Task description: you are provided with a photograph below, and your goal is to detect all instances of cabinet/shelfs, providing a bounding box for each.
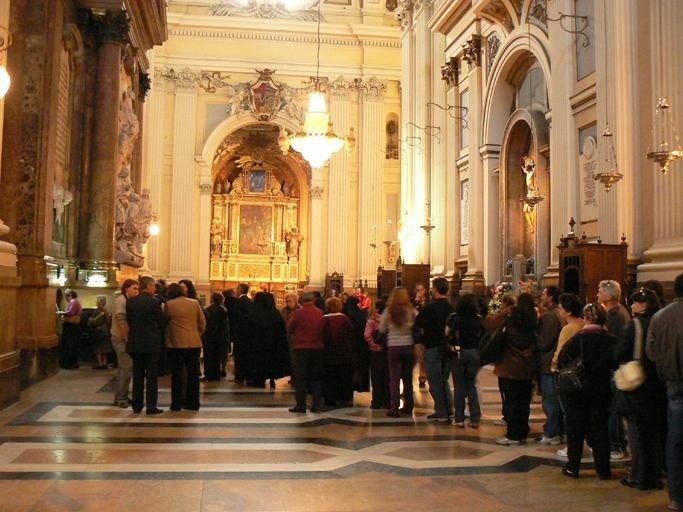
[555,216,628,309]
[377,266,396,300]
[325,271,344,296]
[395,256,430,301]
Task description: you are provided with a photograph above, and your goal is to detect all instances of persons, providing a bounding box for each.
[519,161,537,234]
[200,278,484,429]
[58,275,207,415]
[115,166,160,252]
[484,273,683,512]
[52,189,73,224]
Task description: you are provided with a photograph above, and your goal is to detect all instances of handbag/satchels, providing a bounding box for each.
[372,328,387,344]
[460,317,504,363]
[612,360,645,392]
[551,361,591,414]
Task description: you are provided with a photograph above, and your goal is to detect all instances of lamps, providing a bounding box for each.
[519,0,545,211]
[0,24,13,98]
[646,0,683,175]
[149,210,160,236]
[278,0,355,170]
[592,0,624,192]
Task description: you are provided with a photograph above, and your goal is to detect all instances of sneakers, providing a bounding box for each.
[556,447,682,510]
[491,416,568,445]
[113,397,201,415]
[199,369,277,389]
[54,358,109,372]
[415,382,427,392]
[288,400,481,429]
